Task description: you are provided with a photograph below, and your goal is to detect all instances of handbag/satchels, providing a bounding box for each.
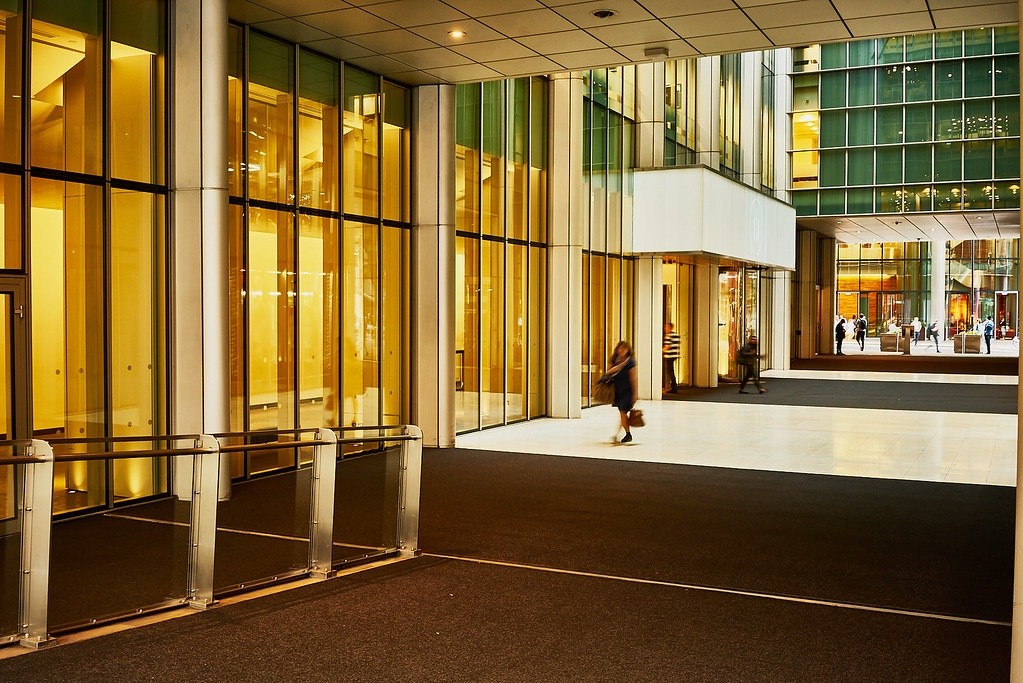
[628,408,645,427]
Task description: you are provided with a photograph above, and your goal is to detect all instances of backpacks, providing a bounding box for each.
[736,347,749,365]
[985,323,993,336]
[859,318,867,329]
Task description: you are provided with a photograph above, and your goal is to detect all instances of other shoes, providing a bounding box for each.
[759,388,768,394]
[738,390,749,394]
[837,352,845,356]
[622,432,632,442]
[666,389,677,394]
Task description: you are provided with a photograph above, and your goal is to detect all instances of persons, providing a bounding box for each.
[889,319,904,332]
[950,312,994,354]
[929,320,941,353]
[999,317,1006,341]
[599,341,639,443]
[661,323,680,394]
[738,336,768,394]
[835,311,867,355]
[909,317,922,349]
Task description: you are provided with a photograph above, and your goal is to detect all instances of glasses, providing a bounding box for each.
[751,338,757,340]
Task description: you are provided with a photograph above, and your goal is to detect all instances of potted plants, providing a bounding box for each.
[880,328,904,352]
[954,329,982,353]
